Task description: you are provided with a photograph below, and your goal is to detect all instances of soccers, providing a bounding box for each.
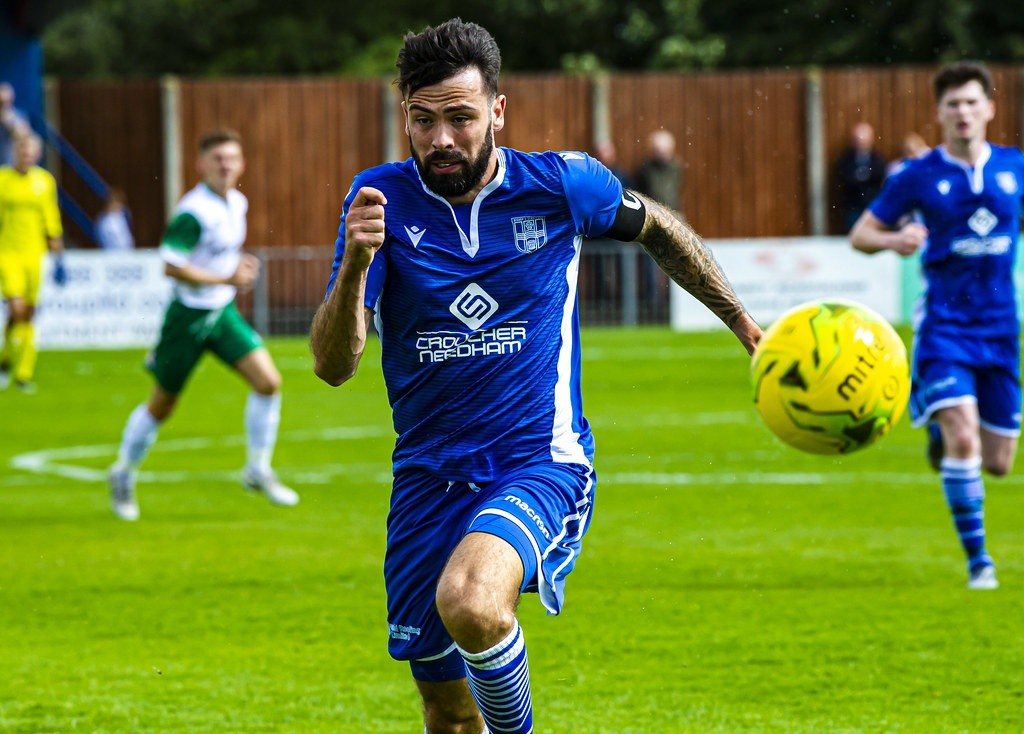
[749,296,913,456]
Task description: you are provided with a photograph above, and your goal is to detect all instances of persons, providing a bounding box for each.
[828,121,892,235]
[306,16,767,734]
[587,128,688,313]
[847,61,1024,591]
[102,127,300,522]
[884,133,934,229]
[0,133,66,390]
[0,82,34,163]
[97,197,135,248]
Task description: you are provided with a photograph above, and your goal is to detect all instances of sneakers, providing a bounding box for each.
[243,468,298,507]
[105,464,143,523]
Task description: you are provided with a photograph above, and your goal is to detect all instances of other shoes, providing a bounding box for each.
[926,421,944,471]
[970,567,999,590]
